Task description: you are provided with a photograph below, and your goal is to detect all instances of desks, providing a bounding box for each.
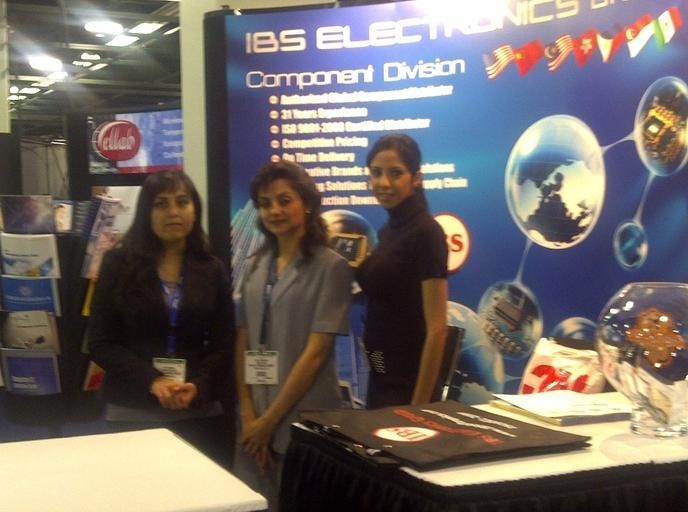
[1,426,268,512]
[289,392,688,508]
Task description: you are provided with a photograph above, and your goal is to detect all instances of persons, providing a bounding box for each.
[88,170,236,470]
[355,134,448,411]
[234,160,352,512]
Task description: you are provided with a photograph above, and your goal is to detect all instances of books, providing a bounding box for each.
[492,389,632,427]
[0,185,142,397]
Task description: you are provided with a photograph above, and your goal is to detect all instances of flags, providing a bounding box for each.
[482,7,683,78]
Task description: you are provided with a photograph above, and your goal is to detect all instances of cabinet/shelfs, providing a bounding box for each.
[56,194,92,394]
